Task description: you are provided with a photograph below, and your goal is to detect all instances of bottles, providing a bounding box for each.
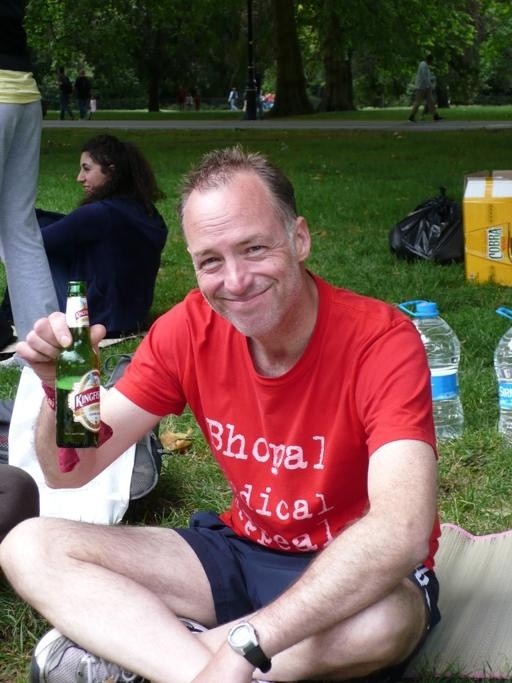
[53,279,102,450]
[493,324,512,441]
[413,300,466,442]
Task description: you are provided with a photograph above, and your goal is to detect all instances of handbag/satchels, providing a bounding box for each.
[0,354,168,521]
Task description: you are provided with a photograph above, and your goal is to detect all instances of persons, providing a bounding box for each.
[408,55,444,124]
[0,135,168,352]
[0,142,442,683]
[0,0,61,367]
[74,69,93,120]
[176,84,277,113]
[56,67,75,120]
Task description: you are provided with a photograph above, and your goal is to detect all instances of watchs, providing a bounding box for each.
[227,621,272,673]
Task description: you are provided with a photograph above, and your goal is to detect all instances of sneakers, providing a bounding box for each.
[29,614,208,683]
[408,115,417,123]
[433,114,446,123]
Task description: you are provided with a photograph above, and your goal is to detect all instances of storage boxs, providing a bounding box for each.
[463,166,510,289]
